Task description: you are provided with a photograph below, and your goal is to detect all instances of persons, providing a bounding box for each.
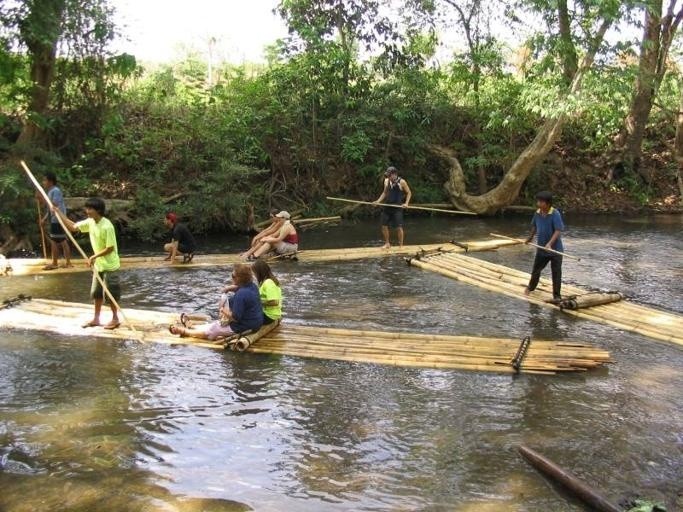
[524,191,565,300]
[50,198,121,330]
[180,260,282,327]
[246,210,298,263]
[239,209,280,258]
[169,263,264,341]
[163,212,197,264]
[34,171,73,271]
[372,166,411,250]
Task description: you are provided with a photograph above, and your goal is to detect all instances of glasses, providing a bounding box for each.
[270,215,275,219]
[231,273,238,279]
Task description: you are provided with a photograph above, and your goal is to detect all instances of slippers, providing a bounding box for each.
[104,322,120,330]
[41,264,57,270]
[81,322,104,329]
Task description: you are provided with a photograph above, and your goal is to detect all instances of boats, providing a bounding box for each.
[0,239,536,277]
[0,294,619,377]
[403,247,682,346]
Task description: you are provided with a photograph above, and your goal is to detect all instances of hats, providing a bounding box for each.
[384,166,397,179]
[165,212,177,222]
[275,211,291,219]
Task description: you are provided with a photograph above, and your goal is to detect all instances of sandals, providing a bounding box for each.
[181,254,193,265]
[181,312,186,325]
[169,325,186,338]
[246,253,260,261]
